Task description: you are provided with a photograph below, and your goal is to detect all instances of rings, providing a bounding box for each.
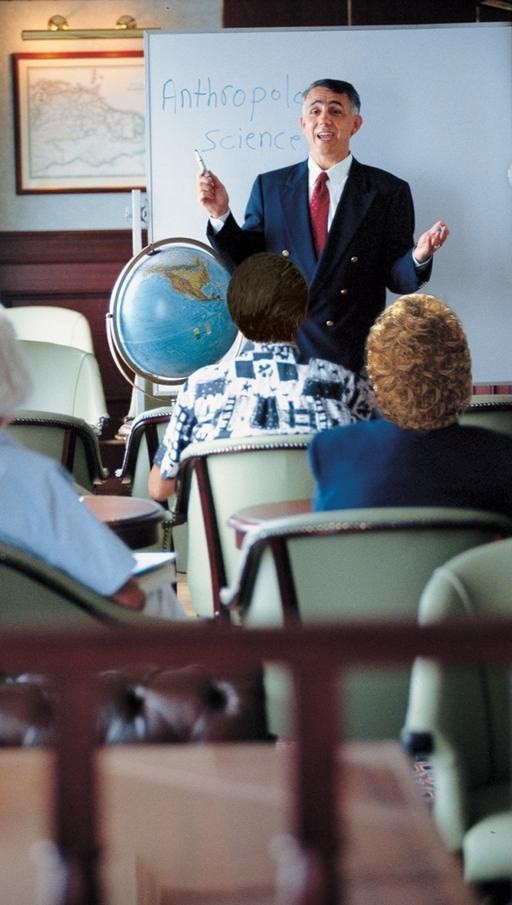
[436,245,441,249]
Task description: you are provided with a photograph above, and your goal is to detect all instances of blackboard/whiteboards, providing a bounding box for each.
[143,21,512,397]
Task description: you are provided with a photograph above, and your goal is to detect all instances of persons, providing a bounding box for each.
[308,295,512,513]
[198,79,447,374]
[2,305,231,747]
[146,251,380,501]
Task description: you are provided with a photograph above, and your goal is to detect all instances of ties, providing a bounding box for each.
[309,173,330,258]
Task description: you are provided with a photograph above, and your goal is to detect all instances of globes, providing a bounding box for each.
[104,238,238,404]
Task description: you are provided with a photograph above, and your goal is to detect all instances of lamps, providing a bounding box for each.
[22,15,162,41]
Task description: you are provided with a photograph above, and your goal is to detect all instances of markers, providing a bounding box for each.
[195,149,215,188]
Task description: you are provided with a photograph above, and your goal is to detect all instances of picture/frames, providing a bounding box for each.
[11,50,146,195]
